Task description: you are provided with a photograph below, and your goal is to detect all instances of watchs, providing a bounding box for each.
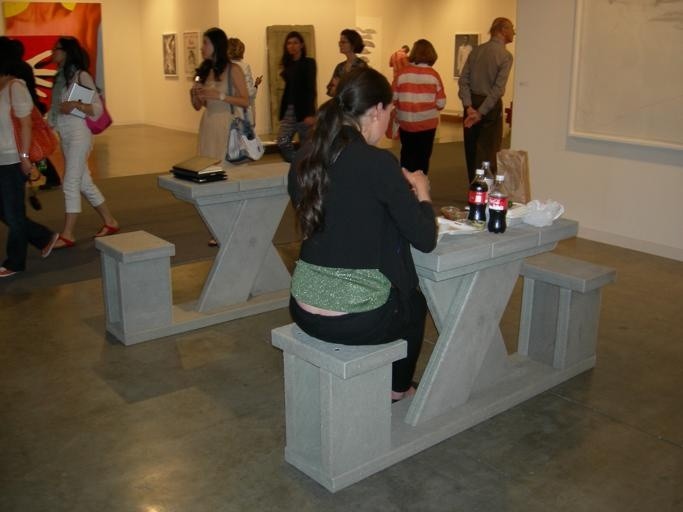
[220,93,226,102]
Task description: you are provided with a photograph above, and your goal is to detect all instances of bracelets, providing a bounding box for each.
[19,152,29,158]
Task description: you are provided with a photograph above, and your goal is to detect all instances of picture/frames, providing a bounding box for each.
[454,33,480,79]
[163,33,180,77]
[182,32,200,78]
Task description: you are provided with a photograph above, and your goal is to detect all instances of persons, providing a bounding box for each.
[227,39,263,127]
[458,18,515,185]
[457,36,472,75]
[390,46,409,78]
[10,40,62,190]
[0,37,59,277]
[190,28,249,246]
[277,32,317,162]
[287,68,437,403]
[47,37,119,249]
[327,29,368,97]
[391,40,446,174]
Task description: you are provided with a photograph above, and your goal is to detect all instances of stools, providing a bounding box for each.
[271,322,409,493]
[519,251,617,384]
[95,228,176,347]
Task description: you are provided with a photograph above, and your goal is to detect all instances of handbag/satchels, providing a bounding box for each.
[81,93,115,138]
[7,79,63,163]
[225,116,268,163]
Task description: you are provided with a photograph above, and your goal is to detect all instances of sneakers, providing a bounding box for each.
[40,231,61,259]
[0,266,20,277]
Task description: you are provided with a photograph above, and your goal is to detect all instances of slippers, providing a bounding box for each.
[51,236,76,251]
[89,220,120,238]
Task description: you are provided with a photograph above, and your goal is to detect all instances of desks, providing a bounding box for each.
[283,202,596,494]
[106,163,296,347]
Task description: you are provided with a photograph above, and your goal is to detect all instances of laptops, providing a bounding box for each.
[68,84,94,118]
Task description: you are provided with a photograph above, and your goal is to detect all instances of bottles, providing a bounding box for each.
[467,160,508,234]
